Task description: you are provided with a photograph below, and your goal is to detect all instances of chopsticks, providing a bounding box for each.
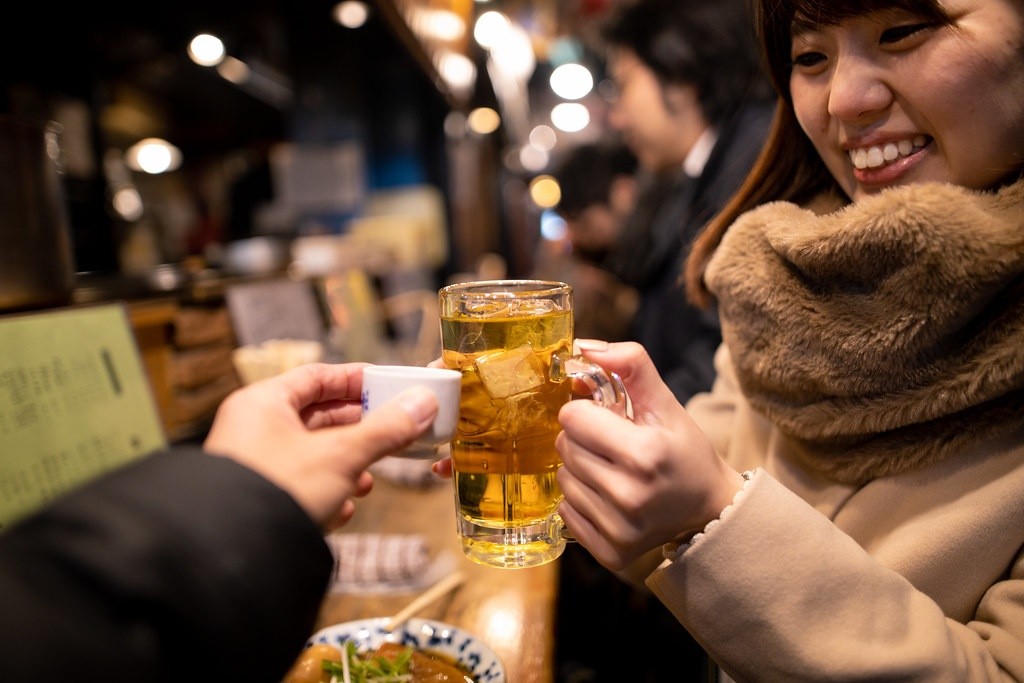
[382,571,475,634]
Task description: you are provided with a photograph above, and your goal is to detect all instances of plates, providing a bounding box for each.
[304,614,506,683]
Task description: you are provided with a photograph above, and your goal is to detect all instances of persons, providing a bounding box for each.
[425,0,1024,683]
[555,1,783,682]
[0,360,439,683]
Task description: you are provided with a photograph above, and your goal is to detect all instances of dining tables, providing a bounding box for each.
[95,277,562,683]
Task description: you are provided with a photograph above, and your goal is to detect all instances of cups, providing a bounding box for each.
[359,365,463,460]
[438,279,629,570]
